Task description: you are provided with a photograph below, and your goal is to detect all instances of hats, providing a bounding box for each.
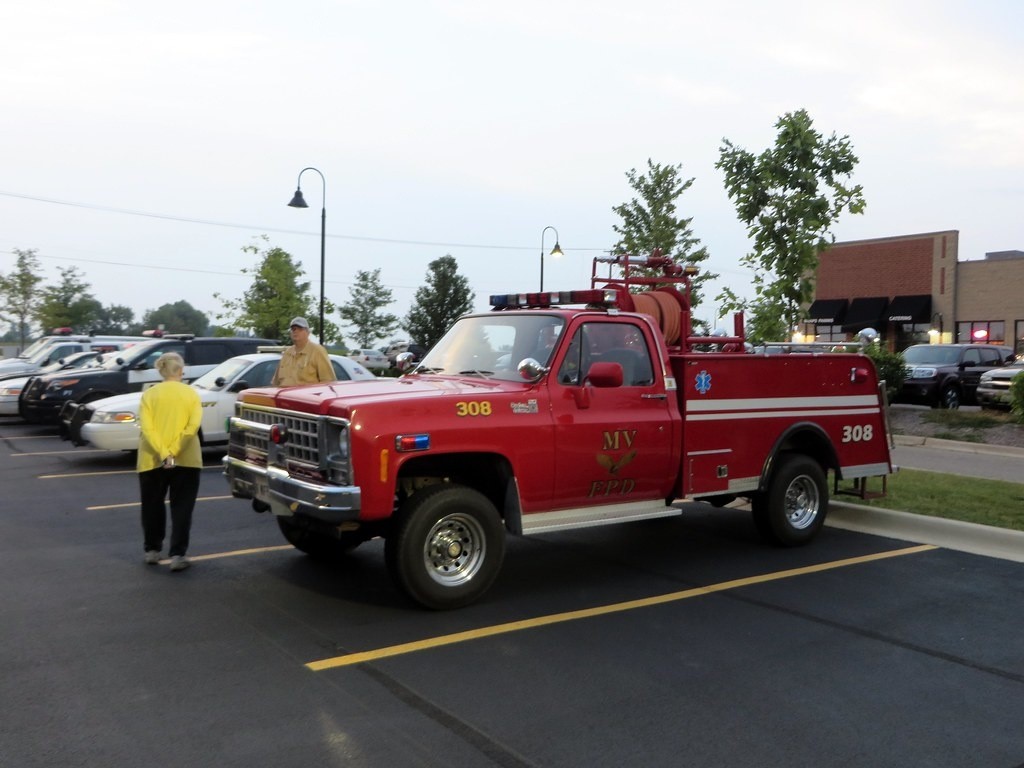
[290,317,310,328]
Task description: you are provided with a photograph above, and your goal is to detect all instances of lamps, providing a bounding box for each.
[927,312,944,336]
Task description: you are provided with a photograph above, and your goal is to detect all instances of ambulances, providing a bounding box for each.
[220,311,900,611]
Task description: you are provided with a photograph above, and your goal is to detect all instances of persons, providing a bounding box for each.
[137,352,203,570]
[534,334,590,385]
[271,316,337,387]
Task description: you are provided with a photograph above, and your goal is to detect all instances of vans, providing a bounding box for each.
[881,344,1015,410]
[17,333,287,425]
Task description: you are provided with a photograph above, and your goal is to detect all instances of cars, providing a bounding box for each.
[382,343,427,363]
[56,352,377,452]
[342,349,390,371]
[976,354,1024,414]
[745,346,786,355]
[0,336,161,415]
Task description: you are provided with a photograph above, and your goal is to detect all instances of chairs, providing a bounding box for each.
[601,346,643,384]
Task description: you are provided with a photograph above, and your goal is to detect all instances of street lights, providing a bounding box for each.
[287,168,326,345]
[540,227,564,292]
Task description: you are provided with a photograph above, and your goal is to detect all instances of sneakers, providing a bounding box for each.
[143,550,160,564]
[170,556,190,571]
